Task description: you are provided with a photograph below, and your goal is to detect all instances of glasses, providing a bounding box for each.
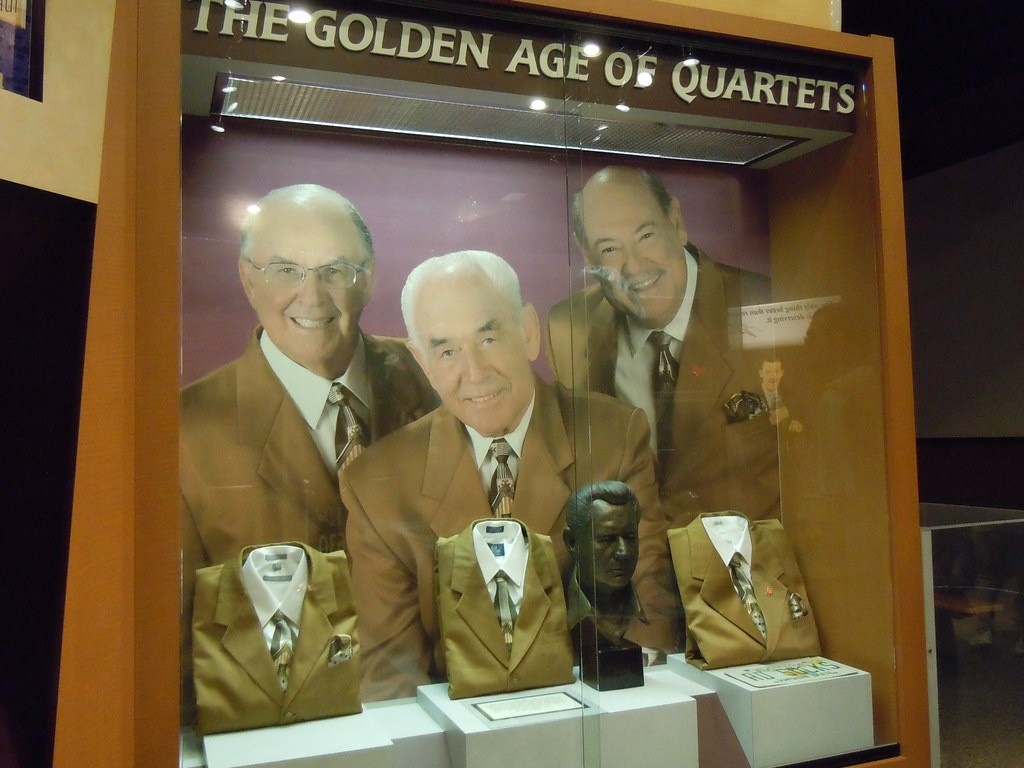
[245,254,373,292]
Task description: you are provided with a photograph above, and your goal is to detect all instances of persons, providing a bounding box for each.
[337,249,679,706]
[544,161,782,525]
[175,183,444,730]
[565,480,650,645]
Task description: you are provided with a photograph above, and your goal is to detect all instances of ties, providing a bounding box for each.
[330,385,368,473]
[488,441,516,519]
[734,553,769,640]
[495,575,517,647]
[270,608,297,687]
[653,329,679,476]
